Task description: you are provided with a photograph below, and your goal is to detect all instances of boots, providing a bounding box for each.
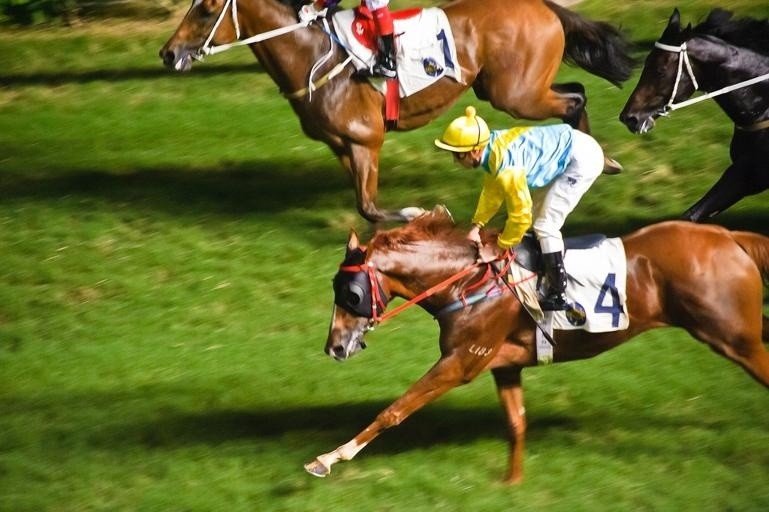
[533,251,571,311]
[378,32,398,78]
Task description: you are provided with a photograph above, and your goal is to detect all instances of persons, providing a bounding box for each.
[297,0,397,79]
[434,106,606,312]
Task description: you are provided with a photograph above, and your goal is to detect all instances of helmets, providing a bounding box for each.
[433,107,492,152]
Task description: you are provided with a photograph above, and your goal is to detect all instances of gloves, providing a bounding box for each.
[299,4,319,21]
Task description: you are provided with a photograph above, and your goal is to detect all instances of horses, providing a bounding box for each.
[158,1,643,222]
[618,5,768,223]
[301,200,769,489]
